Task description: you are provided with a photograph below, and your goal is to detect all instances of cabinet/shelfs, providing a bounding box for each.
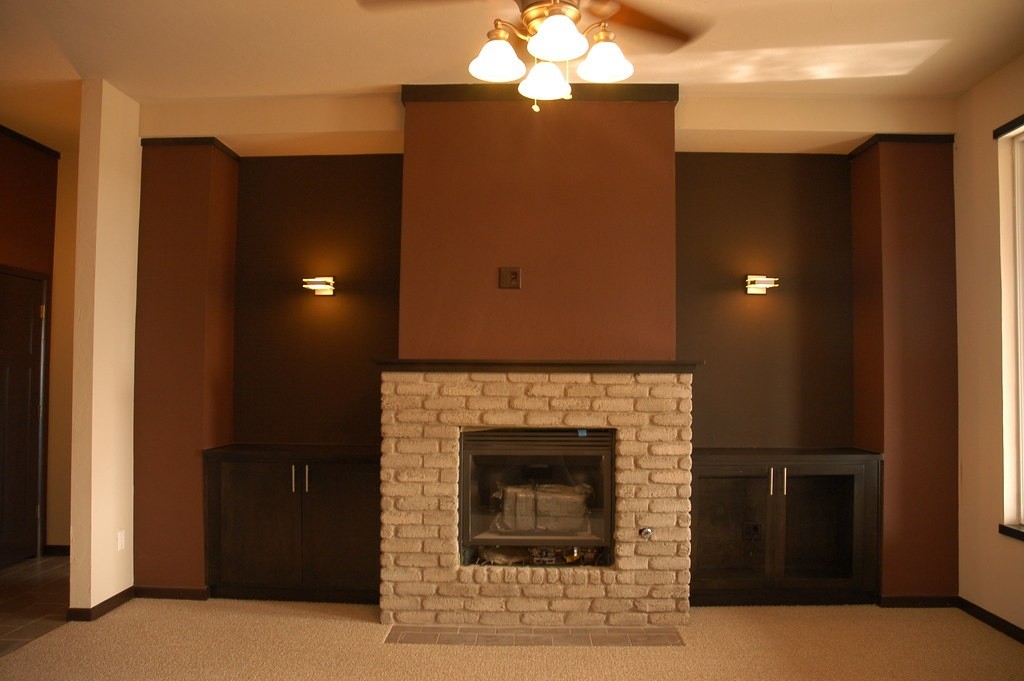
[689,448,883,607]
[204,444,380,604]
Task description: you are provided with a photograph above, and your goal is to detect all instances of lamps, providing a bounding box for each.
[303,277,335,295]
[469,0,634,112]
[746,275,779,294]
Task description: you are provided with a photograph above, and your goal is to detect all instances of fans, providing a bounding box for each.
[356,0,716,56]
